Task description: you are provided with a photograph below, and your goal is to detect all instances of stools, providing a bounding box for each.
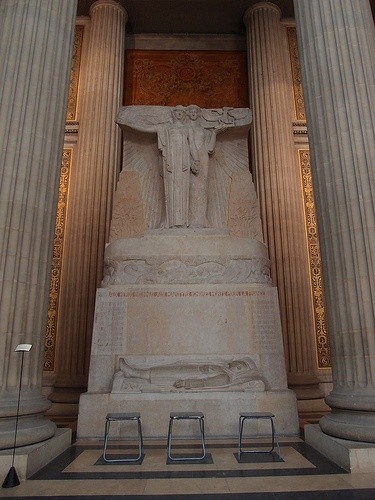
[238,411,282,461]
[166,411,206,460]
[101,412,145,461]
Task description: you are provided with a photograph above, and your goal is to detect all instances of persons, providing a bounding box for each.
[118,358,250,388]
[157,105,200,229]
[185,104,217,230]
